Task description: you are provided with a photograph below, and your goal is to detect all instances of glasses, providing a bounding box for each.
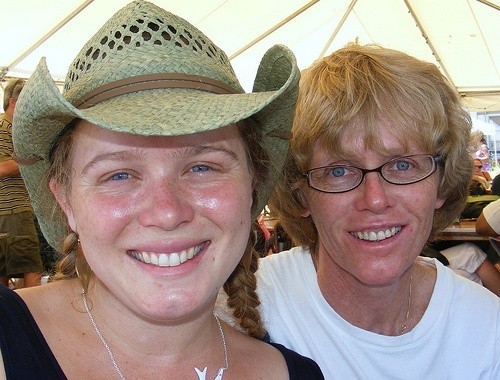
[302,151,444,194]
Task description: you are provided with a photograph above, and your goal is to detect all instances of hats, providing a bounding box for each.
[13,0,302,258]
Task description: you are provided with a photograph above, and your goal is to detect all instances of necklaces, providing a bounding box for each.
[399,265,413,335]
[81,288,229,380]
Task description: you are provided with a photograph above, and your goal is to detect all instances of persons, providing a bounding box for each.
[475,198,500,236]
[461,130,500,219]
[213,46,500,380]
[0,2,324,380]
[1,79,42,289]
[420,239,500,297]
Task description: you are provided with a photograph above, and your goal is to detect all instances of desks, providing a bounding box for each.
[263,217,292,253]
[430,219,500,243]
